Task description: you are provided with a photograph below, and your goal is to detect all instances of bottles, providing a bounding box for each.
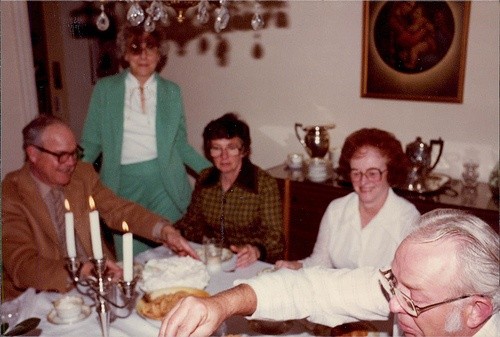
[462,159,480,187]
[489,162,500,199]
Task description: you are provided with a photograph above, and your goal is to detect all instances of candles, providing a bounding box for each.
[89,195,103,259]
[65,199,76,258]
[122,222,133,282]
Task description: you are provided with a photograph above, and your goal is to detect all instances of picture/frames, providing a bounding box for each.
[361,0,471,103]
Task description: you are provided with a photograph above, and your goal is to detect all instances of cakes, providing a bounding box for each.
[142,253,208,294]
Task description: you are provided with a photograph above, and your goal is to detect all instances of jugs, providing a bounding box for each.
[294,122,335,159]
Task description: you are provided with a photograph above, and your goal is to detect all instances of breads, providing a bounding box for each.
[140,290,186,319]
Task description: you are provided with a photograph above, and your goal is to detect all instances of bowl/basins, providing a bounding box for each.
[136,286,211,329]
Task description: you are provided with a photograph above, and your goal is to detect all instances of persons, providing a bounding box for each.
[0,114,202,304]
[76,18,214,262]
[170,110,285,268]
[157,208,500,337]
[274,128,421,271]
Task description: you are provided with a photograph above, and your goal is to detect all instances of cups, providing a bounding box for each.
[204,238,223,277]
[0,298,21,331]
[303,158,333,182]
[52,294,84,320]
[284,153,304,169]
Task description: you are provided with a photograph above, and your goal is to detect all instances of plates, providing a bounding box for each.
[195,246,233,264]
[135,256,210,299]
[59,325,133,337]
[47,305,91,325]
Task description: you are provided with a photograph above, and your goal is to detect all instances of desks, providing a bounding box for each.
[0,241,393,337]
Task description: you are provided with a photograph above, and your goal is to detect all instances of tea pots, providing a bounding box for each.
[405,136,443,190]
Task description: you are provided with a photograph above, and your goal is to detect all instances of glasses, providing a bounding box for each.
[378,268,484,317]
[350,166,383,182]
[210,145,243,157]
[130,45,159,56]
[31,143,84,163]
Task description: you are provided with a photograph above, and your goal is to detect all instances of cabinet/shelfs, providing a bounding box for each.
[265,163,500,261]
